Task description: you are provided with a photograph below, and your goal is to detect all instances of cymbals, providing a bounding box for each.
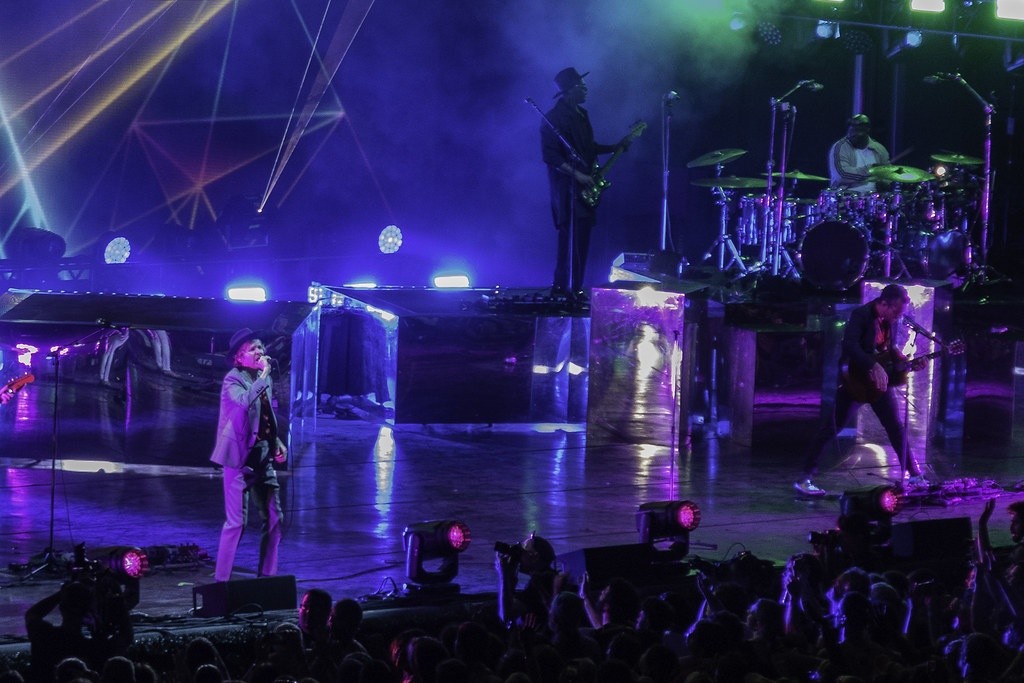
[865,165,936,183]
[688,176,776,189]
[762,171,831,181]
[686,148,749,169]
[931,154,987,165]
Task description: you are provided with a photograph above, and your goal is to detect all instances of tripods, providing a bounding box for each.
[14,327,107,581]
[867,73,1002,286]
[693,79,814,283]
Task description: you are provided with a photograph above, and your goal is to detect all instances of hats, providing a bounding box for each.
[552,67,590,99]
[225,328,264,357]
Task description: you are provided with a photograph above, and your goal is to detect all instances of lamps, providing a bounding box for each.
[840,486,904,560]
[636,500,702,570]
[402,520,471,598]
[216,192,278,243]
[96,230,132,264]
[72,546,149,622]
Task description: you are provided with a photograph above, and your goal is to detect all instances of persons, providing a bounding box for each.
[539,67,633,307]
[793,284,937,495]
[828,113,892,204]
[0,499,1024,683]
[209,328,289,586]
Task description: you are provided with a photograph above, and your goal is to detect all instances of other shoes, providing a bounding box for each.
[794,480,826,494]
[908,475,929,488]
[574,288,590,301]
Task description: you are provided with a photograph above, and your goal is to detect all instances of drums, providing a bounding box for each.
[739,188,946,294]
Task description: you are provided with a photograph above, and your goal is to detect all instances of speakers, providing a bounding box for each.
[889,517,972,561]
[558,543,651,590]
[190,575,297,617]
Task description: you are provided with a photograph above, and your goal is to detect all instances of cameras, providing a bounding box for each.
[493,541,525,568]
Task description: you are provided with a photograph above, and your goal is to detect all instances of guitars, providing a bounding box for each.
[574,118,648,214]
[0,373,35,406]
[837,339,967,403]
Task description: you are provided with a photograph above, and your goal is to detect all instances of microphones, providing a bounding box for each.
[267,358,277,364]
[923,75,945,84]
[805,84,824,90]
[96,318,120,330]
